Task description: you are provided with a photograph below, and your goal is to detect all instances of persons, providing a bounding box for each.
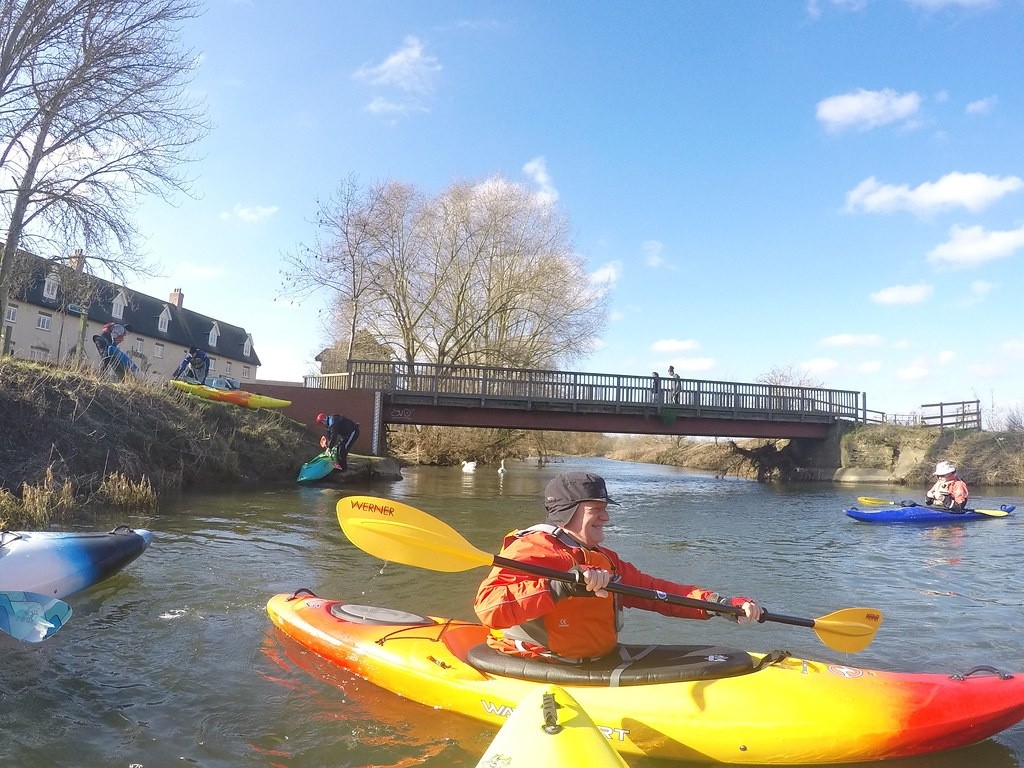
[99,321,147,382]
[668,366,680,404]
[316,414,359,472]
[473,473,764,669]
[652,372,660,403]
[925,462,968,514]
[178,346,209,384]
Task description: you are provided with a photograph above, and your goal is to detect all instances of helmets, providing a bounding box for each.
[316,413,328,428]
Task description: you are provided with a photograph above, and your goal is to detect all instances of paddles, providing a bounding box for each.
[323,434,346,470]
[183,374,229,391]
[336,495,884,655]
[858,497,1010,518]
[0,591,73,643]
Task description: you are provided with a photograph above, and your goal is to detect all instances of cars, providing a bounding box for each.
[207,375,241,391]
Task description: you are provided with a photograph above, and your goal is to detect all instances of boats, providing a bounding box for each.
[298,442,339,482]
[473,683,629,767]
[265,590,1024,767]
[843,504,1017,522]
[1,523,157,610]
[93,333,149,384]
[170,378,293,409]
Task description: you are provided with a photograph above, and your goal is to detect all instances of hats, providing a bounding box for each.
[932,461,955,478]
[122,323,132,333]
[189,345,196,354]
[544,472,619,526]
